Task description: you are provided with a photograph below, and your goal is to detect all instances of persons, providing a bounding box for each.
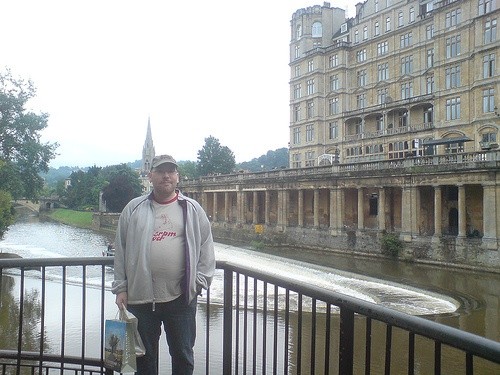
[111,154,216,375]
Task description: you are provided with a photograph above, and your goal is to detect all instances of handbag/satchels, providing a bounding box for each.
[105,301,146,373]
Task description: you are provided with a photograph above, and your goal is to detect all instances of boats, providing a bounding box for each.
[103,247,115,269]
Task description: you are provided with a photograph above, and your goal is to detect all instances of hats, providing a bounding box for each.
[151,155,179,170]
